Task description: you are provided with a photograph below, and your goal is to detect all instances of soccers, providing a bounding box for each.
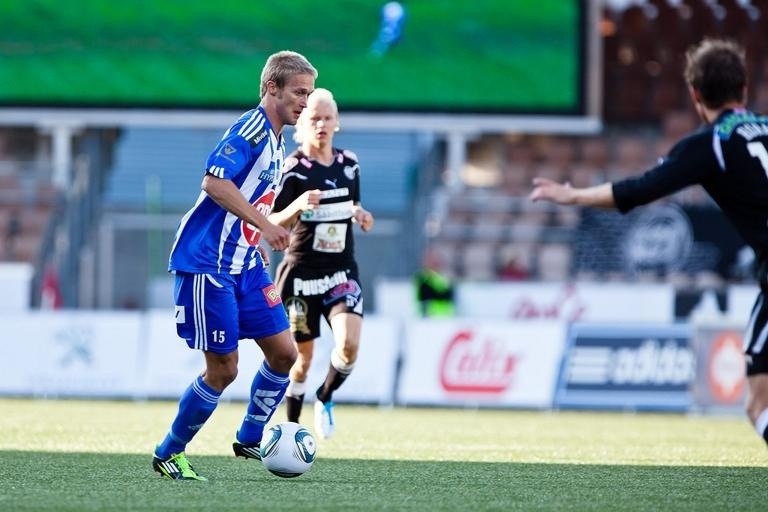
[261,422,316,476]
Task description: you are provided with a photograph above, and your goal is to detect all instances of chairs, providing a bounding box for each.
[1,152,96,309]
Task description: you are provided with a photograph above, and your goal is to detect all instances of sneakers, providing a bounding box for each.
[232,431,262,463]
[151,445,209,483]
[313,394,336,440]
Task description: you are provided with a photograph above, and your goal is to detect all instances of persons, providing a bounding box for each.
[412,253,460,319]
[528,38,767,450]
[499,254,533,282]
[266,86,376,440]
[443,0,767,204]
[1,133,58,263]
[150,48,321,483]
